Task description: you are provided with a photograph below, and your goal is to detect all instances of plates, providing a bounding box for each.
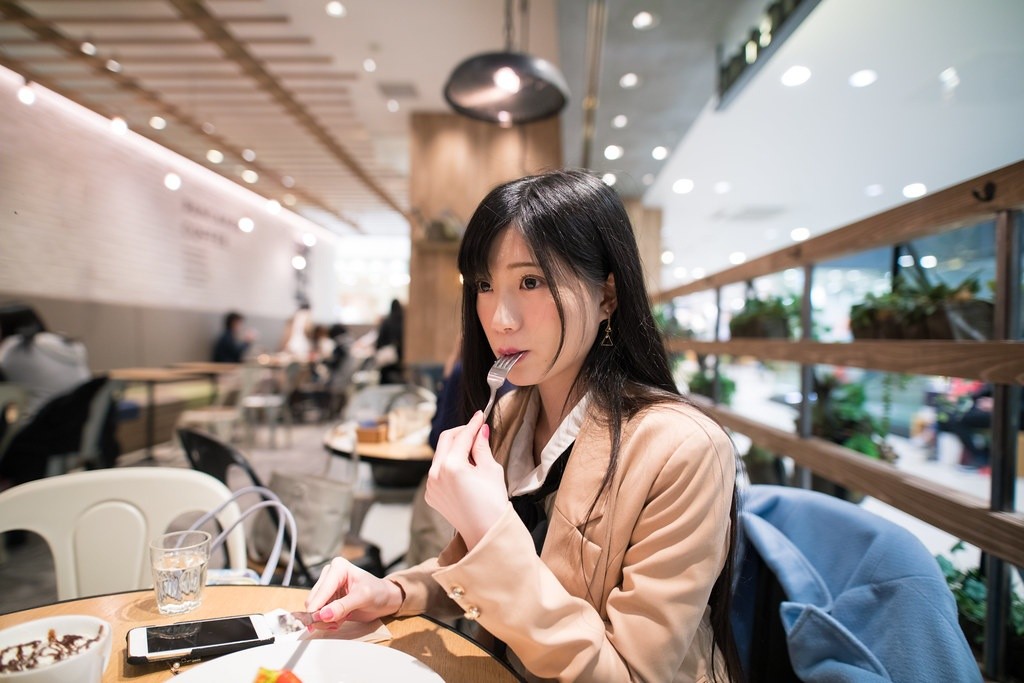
[161,638,445,683]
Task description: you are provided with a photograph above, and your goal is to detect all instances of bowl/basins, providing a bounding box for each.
[0,615,112,683]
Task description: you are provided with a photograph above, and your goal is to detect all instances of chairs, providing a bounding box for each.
[0,370,107,489]
[738,483,955,683]
[167,344,396,465]
[174,425,383,586]
[346,383,438,535]
[0,466,246,611]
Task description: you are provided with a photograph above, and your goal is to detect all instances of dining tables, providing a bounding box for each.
[0,585,530,683]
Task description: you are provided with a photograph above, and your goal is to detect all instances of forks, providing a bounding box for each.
[482,350,525,424]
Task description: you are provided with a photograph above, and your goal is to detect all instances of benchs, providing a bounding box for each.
[117,379,212,455]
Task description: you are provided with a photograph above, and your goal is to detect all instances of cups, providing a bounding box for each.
[148,530,211,616]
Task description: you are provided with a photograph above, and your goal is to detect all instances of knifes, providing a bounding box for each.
[278,609,324,634]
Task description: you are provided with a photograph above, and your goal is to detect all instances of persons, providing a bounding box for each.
[285,294,312,355]
[304,173,738,682]
[211,312,253,363]
[0,305,119,544]
[376,300,405,384]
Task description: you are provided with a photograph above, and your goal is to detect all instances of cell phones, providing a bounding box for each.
[126,613,275,665]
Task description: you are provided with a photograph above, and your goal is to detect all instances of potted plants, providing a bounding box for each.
[689,266,1023,664]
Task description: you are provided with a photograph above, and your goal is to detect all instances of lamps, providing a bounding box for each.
[442,0,570,128]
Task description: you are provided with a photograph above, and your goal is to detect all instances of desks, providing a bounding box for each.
[105,368,213,465]
[322,419,433,536]
[172,359,242,404]
